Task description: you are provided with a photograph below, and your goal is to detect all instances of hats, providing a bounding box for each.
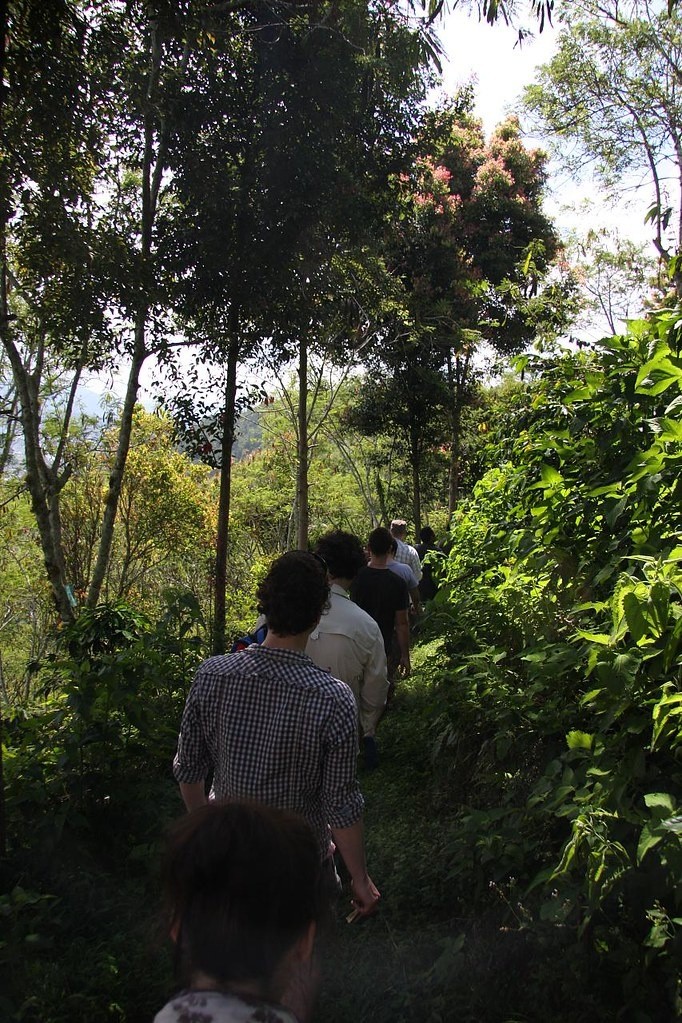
[391,519,407,535]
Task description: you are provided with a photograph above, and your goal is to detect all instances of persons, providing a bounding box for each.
[308,520,447,742]
[174,550,378,950]
[152,802,336,1023]
